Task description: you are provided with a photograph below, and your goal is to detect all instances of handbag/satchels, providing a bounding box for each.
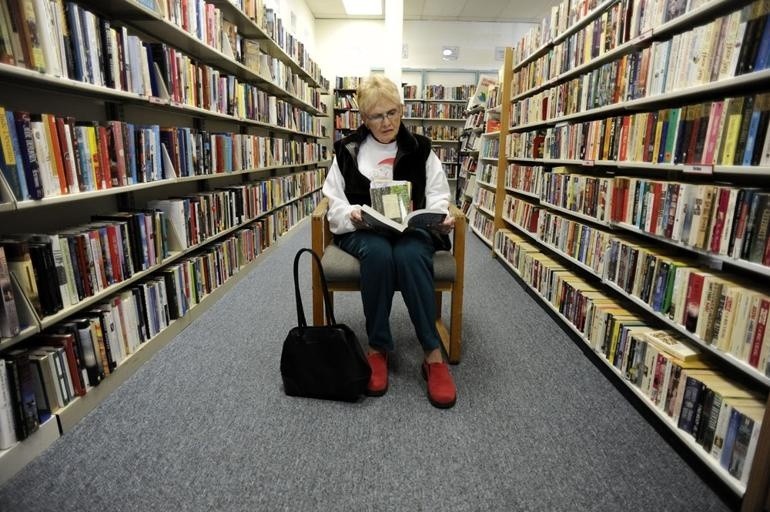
[280,325,371,402]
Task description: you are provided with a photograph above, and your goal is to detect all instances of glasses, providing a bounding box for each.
[365,107,402,122]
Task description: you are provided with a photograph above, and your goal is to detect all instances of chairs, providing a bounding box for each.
[311,198,467,365]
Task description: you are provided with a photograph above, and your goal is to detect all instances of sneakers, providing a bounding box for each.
[364,348,389,398]
[419,359,457,408]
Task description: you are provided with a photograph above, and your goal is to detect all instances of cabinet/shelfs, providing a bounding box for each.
[333,87,469,181]
[458,0,769,511]
[0,1,330,487]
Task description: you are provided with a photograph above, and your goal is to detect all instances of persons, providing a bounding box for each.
[322,74,457,409]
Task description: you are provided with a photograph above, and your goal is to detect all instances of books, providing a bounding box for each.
[1,0,330,449]
[333,77,457,235]
[458,1,770,486]
